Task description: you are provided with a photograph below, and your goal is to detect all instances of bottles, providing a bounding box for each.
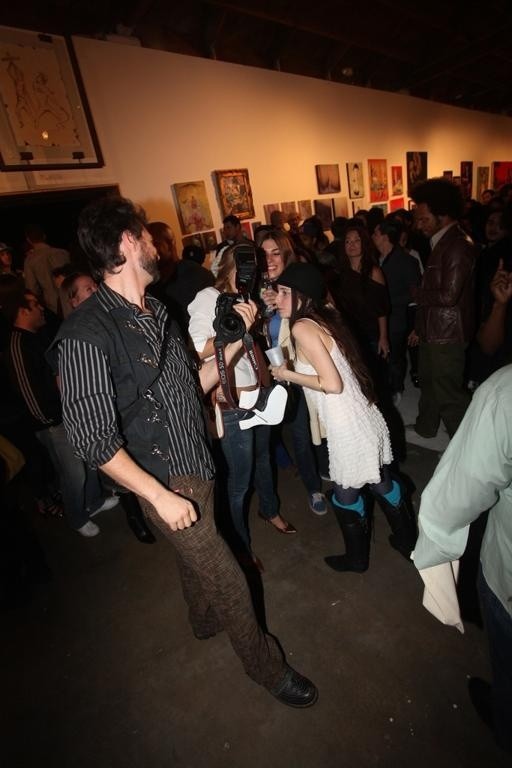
[258,270,274,317]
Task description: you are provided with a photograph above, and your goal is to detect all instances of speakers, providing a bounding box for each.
[0,184,122,267]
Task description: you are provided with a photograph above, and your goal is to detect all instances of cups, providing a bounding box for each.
[264,347,284,368]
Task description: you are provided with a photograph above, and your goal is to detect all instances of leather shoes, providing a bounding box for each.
[242,554,267,576]
[272,664,318,710]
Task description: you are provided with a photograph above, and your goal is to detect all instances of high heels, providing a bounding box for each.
[255,507,298,535]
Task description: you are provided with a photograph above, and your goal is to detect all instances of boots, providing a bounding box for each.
[320,485,378,575]
[376,475,418,564]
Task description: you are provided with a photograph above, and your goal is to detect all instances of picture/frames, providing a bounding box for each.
[408,199,417,211]
[0,22,106,175]
[214,166,258,222]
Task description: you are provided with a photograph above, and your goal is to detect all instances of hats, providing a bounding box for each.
[0,243,11,253]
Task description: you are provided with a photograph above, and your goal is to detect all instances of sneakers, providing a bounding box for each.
[36,497,64,524]
[466,677,495,735]
[88,496,121,518]
[307,492,329,517]
[75,520,102,538]
[122,503,158,544]
